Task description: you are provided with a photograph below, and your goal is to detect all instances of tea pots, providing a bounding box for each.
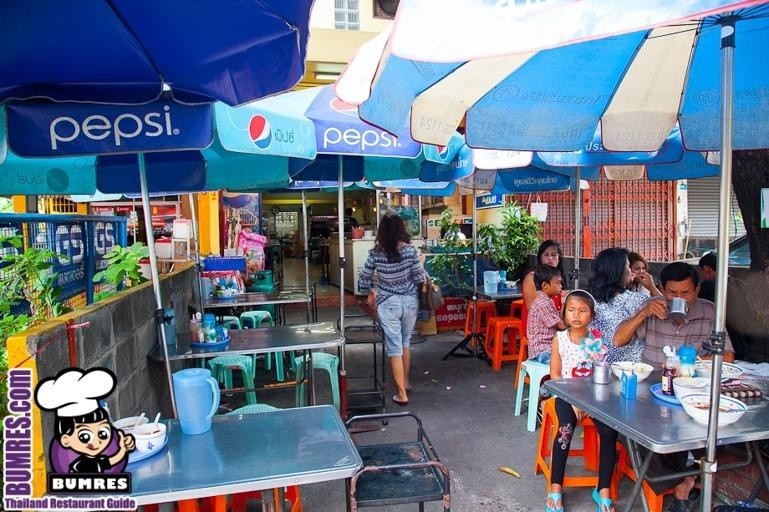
[203,313,216,328]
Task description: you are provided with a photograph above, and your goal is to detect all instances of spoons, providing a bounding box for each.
[725,409,757,413]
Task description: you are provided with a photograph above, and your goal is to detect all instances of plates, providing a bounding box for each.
[217,297,235,300]
[650,383,682,404]
[498,288,519,291]
[128,435,168,464]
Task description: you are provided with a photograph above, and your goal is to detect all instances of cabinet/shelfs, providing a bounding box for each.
[273,281,318,324]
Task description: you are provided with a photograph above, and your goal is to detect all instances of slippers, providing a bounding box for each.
[545,490,564,512]
[392,394,408,407]
[591,486,616,512]
[667,487,701,512]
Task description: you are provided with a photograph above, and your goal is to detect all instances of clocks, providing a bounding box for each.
[271,205,280,215]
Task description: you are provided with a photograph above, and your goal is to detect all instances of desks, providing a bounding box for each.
[545,361,768,511]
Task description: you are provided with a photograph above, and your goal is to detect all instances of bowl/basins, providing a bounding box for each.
[364,230,373,236]
[680,392,748,426]
[506,281,517,289]
[611,362,654,382]
[672,377,708,400]
[131,423,166,454]
[694,360,744,380]
[113,416,149,434]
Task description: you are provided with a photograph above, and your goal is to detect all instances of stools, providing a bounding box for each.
[465,300,689,512]
[82,310,364,512]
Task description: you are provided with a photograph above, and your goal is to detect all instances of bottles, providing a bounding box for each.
[216,325,225,342]
[676,345,698,377]
[198,327,204,343]
[500,270,507,289]
[188,314,198,342]
[215,286,221,297]
[662,346,680,396]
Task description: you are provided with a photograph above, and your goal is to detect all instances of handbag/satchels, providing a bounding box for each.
[417,271,444,314]
[530,195,548,223]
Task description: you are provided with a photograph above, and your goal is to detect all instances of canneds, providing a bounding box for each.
[662,367,677,395]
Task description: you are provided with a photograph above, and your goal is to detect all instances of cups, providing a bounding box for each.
[666,297,689,319]
[594,363,611,384]
[364,230,373,237]
